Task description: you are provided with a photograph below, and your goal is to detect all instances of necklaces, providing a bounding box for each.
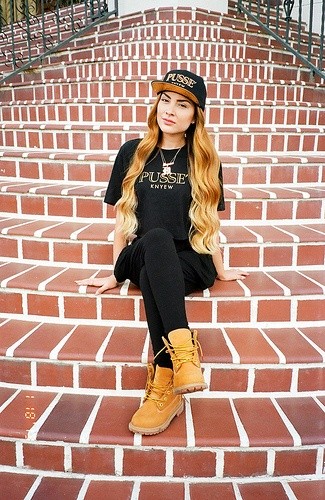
[156,143,187,175]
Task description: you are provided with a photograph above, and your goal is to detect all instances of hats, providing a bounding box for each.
[151,70,206,111]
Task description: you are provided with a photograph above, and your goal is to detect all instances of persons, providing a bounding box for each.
[73,69,250,436]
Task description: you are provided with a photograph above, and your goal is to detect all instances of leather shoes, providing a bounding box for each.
[128,363,184,435]
[160,328,207,395]
